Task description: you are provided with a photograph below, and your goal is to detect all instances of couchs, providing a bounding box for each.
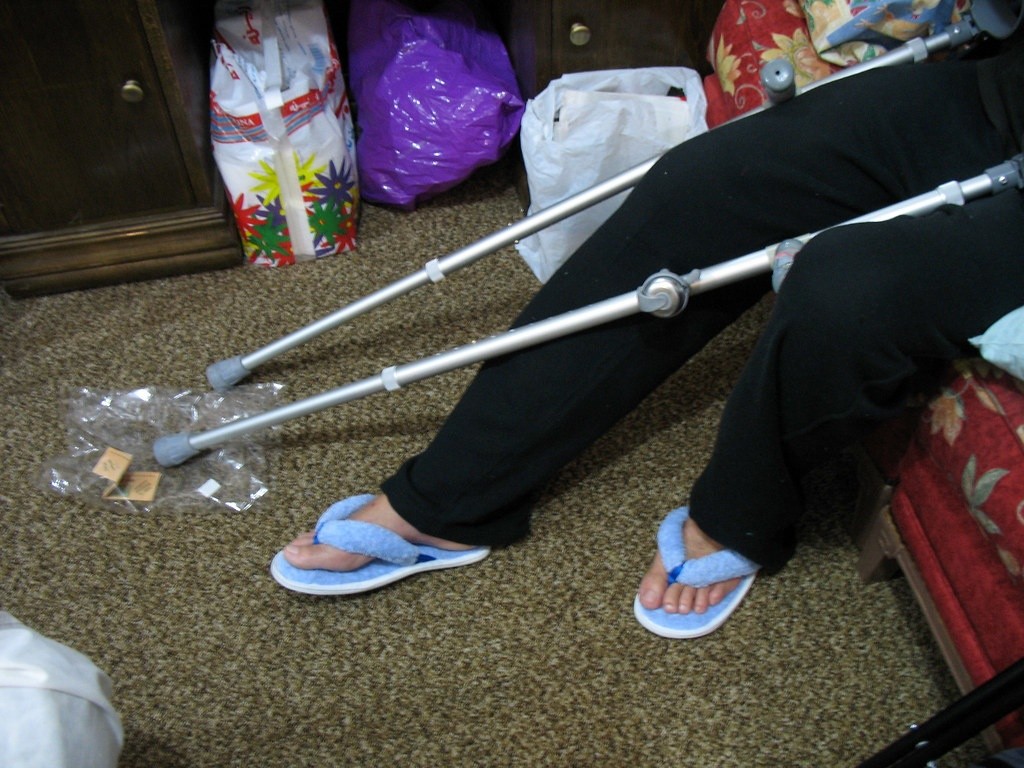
[702,0,1024,768]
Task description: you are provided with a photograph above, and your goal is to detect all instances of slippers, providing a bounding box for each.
[633,506,760,639]
[270,497,491,594]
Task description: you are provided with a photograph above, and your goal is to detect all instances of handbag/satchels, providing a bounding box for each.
[209,0,356,266]
[517,64,709,288]
[335,9,524,209]
[800,1,971,68]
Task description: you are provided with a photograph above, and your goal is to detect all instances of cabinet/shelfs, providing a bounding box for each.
[0,0,244,299]
[508,0,727,104]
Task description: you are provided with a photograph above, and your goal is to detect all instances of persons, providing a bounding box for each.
[270,0,1023,638]
